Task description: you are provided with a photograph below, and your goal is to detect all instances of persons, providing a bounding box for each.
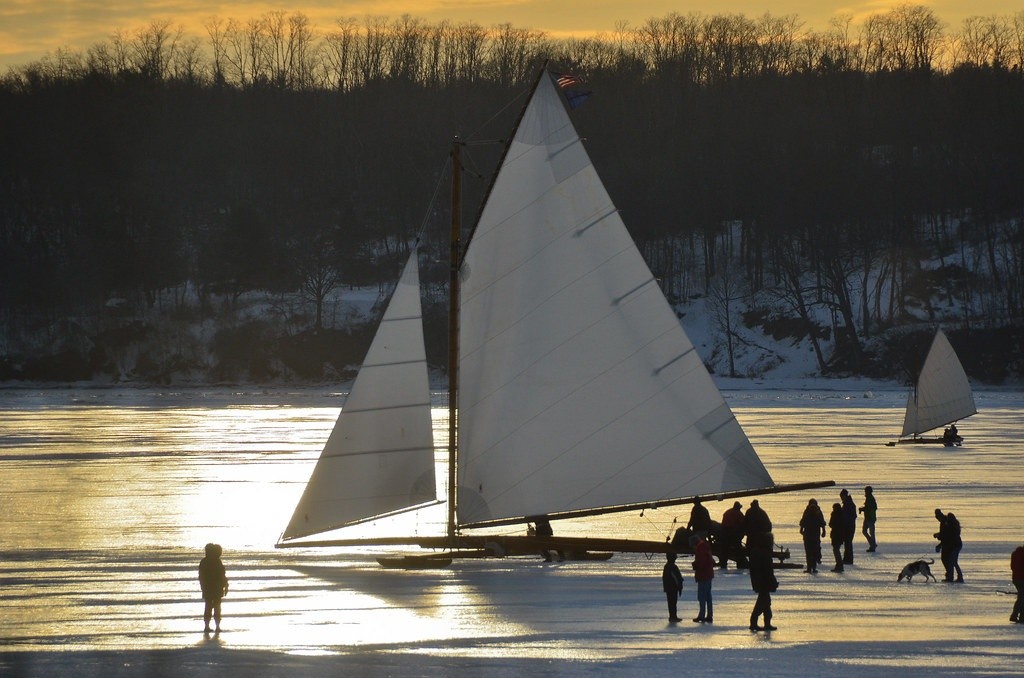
[944,425,962,447]
[933,509,964,582]
[1009,545,1024,624]
[662,551,684,622]
[199,544,229,632]
[800,486,878,573]
[686,496,772,570]
[749,533,779,631]
[689,535,716,623]
[534,514,566,563]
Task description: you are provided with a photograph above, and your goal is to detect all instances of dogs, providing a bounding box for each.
[895,558,937,584]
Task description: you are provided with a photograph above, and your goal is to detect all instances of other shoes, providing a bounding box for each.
[749,624,763,631]
[763,625,778,630]
[677,618,682,621]
[706,618,713,623]
[954,578,964,583]
[669,618,680,622]
[1019,619,1024,623]
[692,617,705,622]
[942,579,953,583]
[1010,617,1018,621]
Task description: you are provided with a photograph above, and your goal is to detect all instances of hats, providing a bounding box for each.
[935,509,943,518]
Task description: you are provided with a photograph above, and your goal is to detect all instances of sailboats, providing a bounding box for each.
[886,325,981,445]
[258,60,839,575]
